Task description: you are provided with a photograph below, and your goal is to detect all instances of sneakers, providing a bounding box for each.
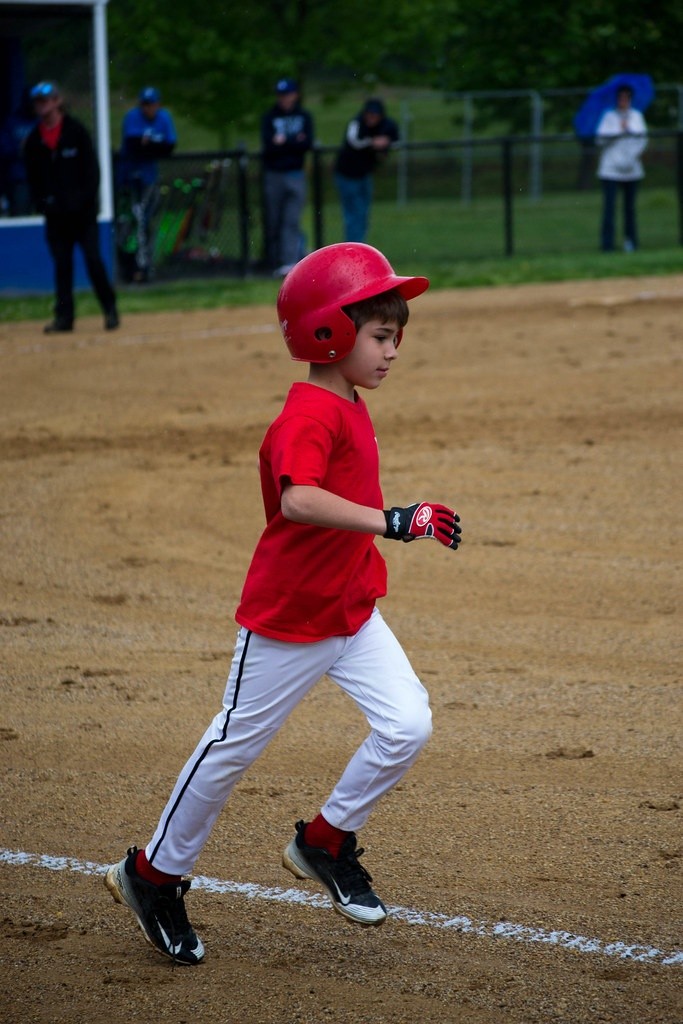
[102,845,205,965]
[281,820,386,924]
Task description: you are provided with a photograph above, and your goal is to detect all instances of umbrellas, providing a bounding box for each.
[574,75,656,139]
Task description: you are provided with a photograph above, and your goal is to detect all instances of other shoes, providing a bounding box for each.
[104,312,118,329]
[280,264,294,276]
[44,322,73,333]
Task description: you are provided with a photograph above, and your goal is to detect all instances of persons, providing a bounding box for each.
[118,88,178,266]
[593,86,650,255]
[103,242,464,963]
[22,82,121,333]
[259,80,316,276]
[329,98,400,242]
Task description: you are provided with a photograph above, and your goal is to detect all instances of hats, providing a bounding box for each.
[275,79,295,95]
[138,87,158,102]
[29,82,57,104]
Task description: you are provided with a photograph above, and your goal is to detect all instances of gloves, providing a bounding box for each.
[383,501,462,552]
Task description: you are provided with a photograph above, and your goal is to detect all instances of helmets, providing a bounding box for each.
[277,241,430,363]
